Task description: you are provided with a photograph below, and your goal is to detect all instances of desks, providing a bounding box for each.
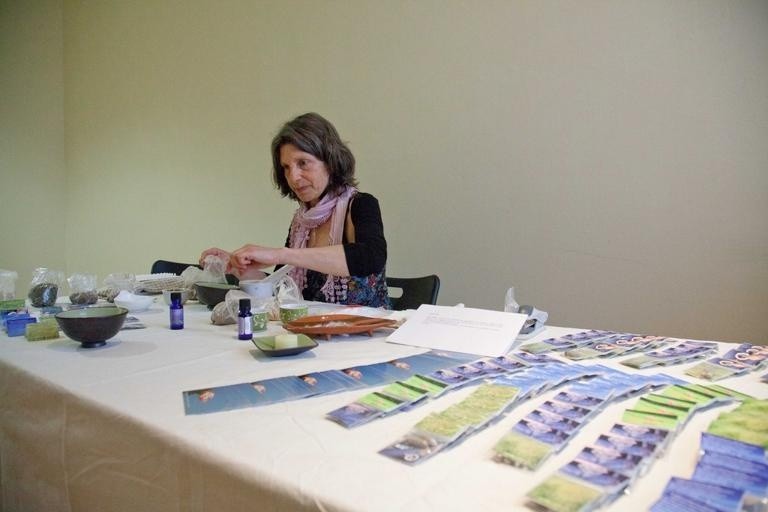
[1,299,767,512]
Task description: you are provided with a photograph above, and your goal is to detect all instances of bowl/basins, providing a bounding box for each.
[196,281,239,309]
[57,307,128,348]
[113,296,156,313]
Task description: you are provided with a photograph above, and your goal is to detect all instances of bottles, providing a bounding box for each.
[169,291,184,330]
[237,299,254,340]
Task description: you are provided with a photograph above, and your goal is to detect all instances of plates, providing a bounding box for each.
[286,313,396,336]
[252,333,317,356]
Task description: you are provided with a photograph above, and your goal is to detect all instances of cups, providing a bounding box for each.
[162,288,196,305]
[280,305,308,327]
[240,278,274,299]
[251,310,266,331]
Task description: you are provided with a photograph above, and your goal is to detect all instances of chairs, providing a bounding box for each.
[152,260,270,289]
[385,274,439,310]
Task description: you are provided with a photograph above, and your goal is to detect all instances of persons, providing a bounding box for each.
[199,112,392,309]
